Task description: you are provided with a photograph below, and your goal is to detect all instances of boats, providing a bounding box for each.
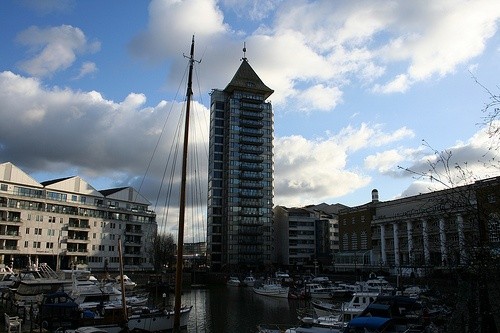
[0,237,467,333]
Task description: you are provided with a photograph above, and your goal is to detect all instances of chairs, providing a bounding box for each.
[5,313,19,331]
[6,315,21,333]
